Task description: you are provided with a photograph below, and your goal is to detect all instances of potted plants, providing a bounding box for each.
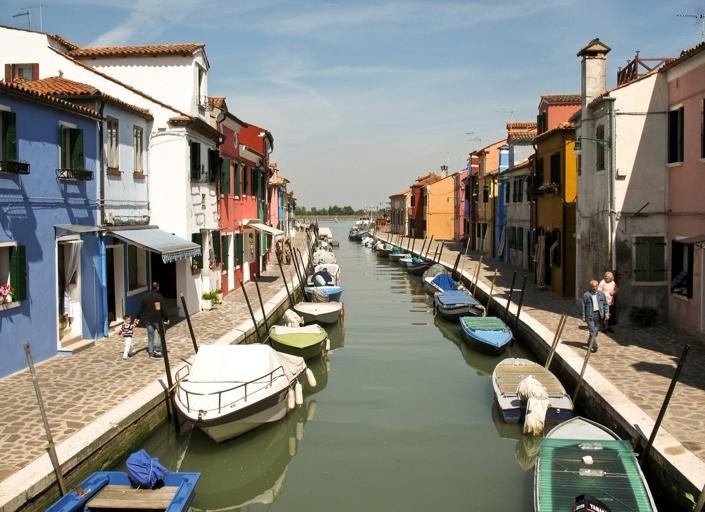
[201,288,223,311]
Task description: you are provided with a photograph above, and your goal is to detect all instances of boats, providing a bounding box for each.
[292,301,344,325]
[458,315,514,354]
[433,289,485,318]
[265,318,330,363]
[41,470,202,512]
[304,248,345,303]
[173,340,317,446]
[491,356,577,425]
[531,414,660,512]
[349,228,472,297]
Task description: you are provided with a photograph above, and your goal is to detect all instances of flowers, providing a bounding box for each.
[0,283,16,303]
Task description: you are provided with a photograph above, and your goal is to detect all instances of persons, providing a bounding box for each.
[118,314,139,360]
[582,280,611,353]
[598,272,618,333]
[276,236,283,265]
[284,240,291,264]
[134,281,170,357]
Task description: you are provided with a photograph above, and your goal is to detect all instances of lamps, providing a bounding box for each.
[572,136,613,155]
